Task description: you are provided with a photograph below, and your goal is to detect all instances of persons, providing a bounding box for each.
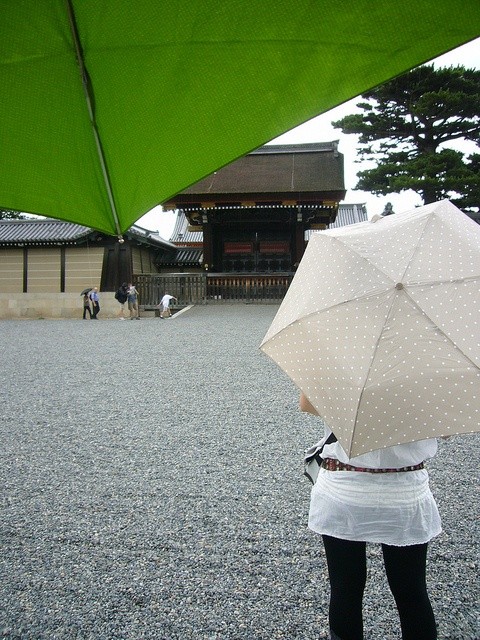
[82,292,92,321]
[127,283,140,321]
[157,293,177,319]
[90,287,101,319]
[114,282,128,322]
[293,386,448,634]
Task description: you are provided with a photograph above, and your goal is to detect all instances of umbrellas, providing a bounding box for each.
[80,288,92,295]
[0,2,478,246]
[259,198,479,455]
